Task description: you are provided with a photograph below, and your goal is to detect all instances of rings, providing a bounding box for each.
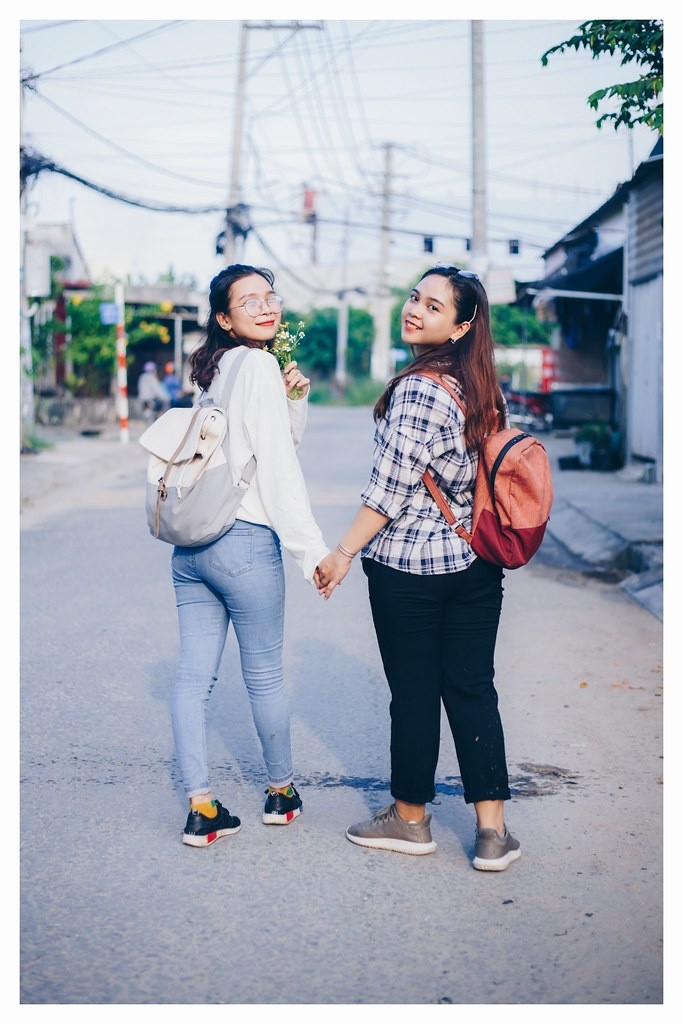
[296,369,300,375]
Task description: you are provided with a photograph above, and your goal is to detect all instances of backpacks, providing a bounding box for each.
[139,348,257,547]
[416,371,553,570]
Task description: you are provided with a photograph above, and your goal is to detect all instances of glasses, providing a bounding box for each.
[228,295,284,318]
[436,262,479,323]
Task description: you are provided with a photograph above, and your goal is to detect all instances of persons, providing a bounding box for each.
[166,262,345,850]
[160,362,187,410]
[314,266,525,874]
[139,362,172,418]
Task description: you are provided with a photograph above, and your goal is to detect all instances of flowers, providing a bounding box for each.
[263,320,306,400]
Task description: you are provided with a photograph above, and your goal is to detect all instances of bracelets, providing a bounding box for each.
[337,543,356,558]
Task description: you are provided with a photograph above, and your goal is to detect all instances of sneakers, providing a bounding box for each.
[182,799,242,848]
[472,823,522,870]
[262,782,303,824]
[345,803,438,855]
